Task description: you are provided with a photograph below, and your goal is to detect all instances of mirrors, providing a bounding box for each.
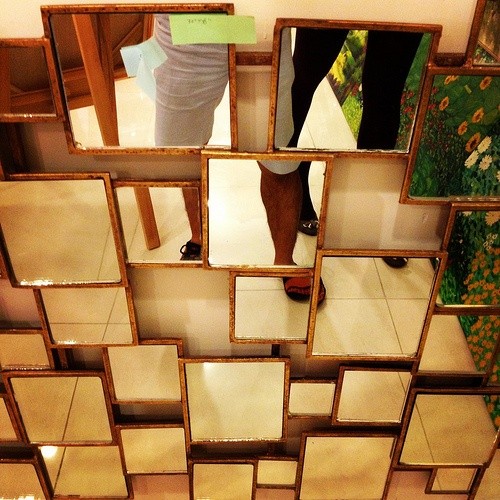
[0,0,500,500]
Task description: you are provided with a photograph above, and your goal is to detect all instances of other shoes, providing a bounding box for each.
[382,258,408,268]
[283,276,326,306]
[298,214,319,236]
[180,240,202,260]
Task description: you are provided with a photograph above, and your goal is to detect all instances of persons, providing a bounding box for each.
[289,26,423,269]
[147,13,329,306]
